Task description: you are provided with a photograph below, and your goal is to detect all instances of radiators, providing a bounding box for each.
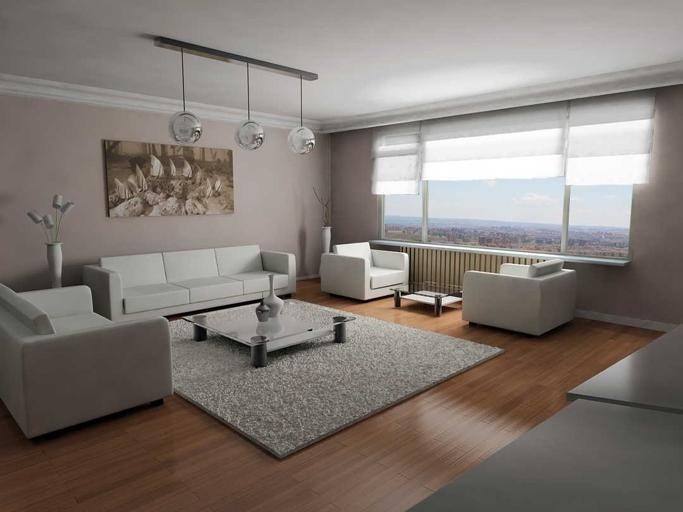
[399,246,547,303]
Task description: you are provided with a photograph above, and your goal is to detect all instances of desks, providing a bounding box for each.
[402,398,683,512]
[567,319,683,414]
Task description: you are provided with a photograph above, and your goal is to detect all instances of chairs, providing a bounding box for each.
[320,242,410,302]
[0,284,172,440]
[464,259,578,340]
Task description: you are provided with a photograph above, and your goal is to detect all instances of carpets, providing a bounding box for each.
[168,306,506,459]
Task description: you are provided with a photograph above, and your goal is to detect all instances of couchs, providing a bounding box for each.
[83,243,296,319]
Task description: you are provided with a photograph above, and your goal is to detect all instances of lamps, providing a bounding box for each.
[152,37,319,156]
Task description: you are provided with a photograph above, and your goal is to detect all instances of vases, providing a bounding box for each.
[263,275,284,317]
[320,226,332,253]
[45,241,64,288]
